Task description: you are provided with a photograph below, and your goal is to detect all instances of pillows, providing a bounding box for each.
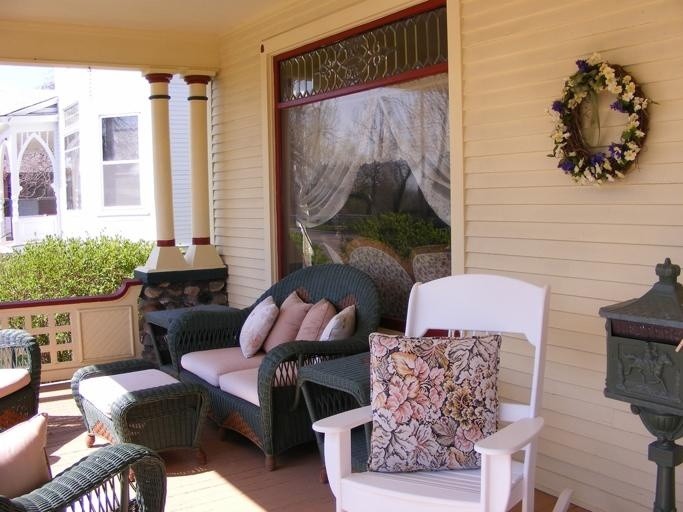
[0,410,53,497]
[239,292,353,367]
[363,327,501,471]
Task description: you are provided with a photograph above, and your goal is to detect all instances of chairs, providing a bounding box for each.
[311,265,575,512]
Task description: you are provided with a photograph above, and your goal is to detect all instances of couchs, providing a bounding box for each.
[168,267,383,469]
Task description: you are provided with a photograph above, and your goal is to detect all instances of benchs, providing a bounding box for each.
[70,358,207,469]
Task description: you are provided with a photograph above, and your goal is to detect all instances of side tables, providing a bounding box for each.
[290,351,383,460]
[146,302,235,377]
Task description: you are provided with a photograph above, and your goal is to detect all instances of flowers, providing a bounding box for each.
[539,51,650,184]
